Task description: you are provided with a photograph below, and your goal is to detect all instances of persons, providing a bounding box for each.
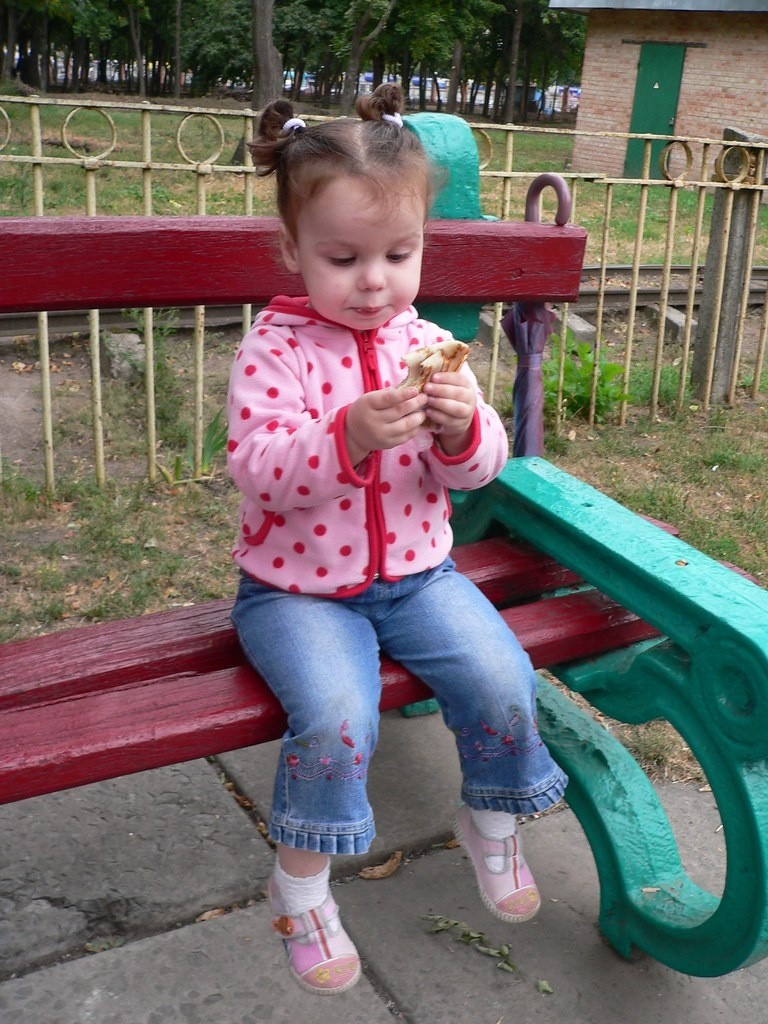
[238,86,572,998]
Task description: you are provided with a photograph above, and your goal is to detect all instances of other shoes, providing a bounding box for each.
[449,794,540,924]
[268,872,360,995]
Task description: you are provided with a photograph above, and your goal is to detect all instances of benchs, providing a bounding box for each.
[2,222,768,979]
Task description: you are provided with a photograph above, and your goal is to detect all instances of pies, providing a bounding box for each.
[398,340,470,433]
